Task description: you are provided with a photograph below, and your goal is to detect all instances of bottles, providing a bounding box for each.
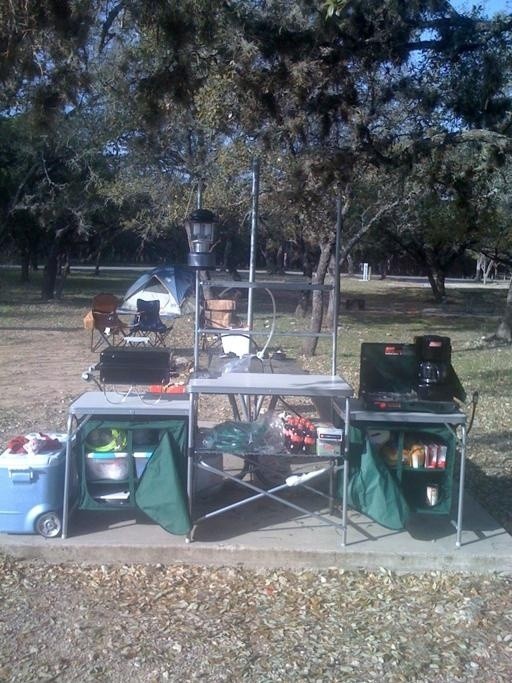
[284,418,315,455]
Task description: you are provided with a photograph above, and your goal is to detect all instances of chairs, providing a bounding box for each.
[200,299,243,352]
[83,293,133,351]
[130,299,167,348]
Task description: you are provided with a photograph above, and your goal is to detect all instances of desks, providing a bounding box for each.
[61,391,198,539]
[186,372,353,546]
[334,397,467,546]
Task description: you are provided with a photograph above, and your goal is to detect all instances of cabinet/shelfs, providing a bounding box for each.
[339,420,456,531]
[77,416,192,536]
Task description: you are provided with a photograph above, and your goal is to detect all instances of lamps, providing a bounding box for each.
[182,209,219,268]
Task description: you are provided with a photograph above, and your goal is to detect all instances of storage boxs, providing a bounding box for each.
[100,345,170,384]
[0,432,78,534]
[83,450,129,480]
[132,445,156,479]
